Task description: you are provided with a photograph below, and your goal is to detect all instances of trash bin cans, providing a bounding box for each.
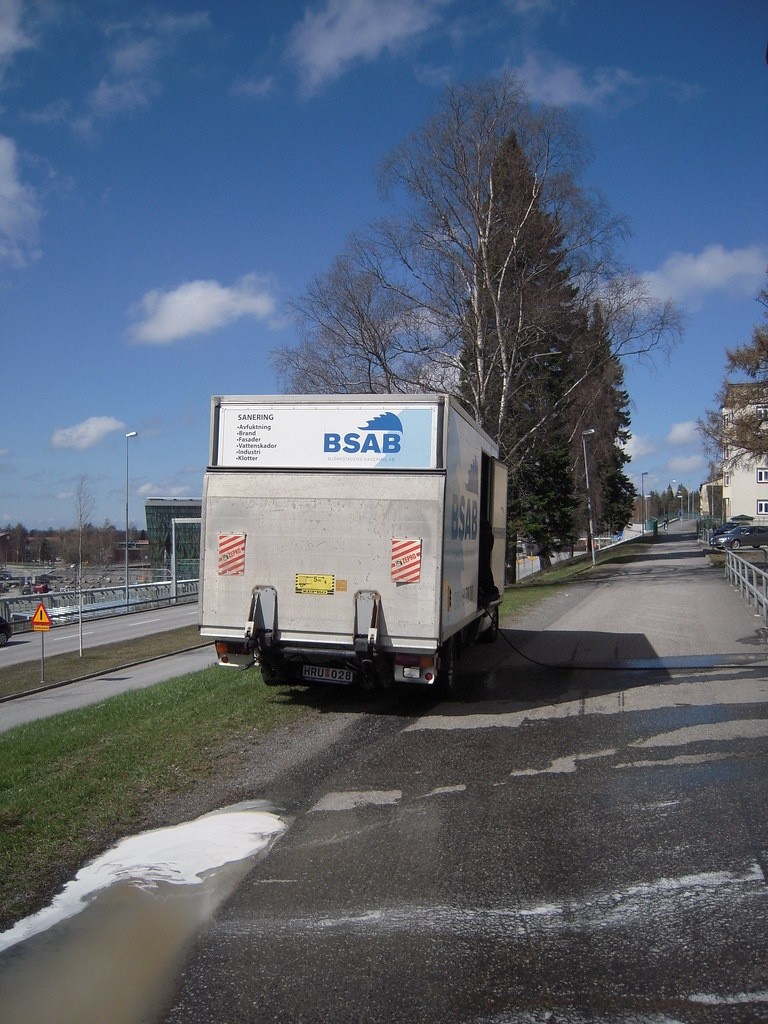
[647,518,658,536]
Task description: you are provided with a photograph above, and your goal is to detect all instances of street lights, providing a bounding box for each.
[123,430,138,611]
[641,471,648,534]
[71,564,76,598]
[676,495,683,531]
[644,493,652,531]
[663,479,676,517]
[579,427,598,566]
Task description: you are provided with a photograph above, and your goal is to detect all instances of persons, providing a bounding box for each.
[477,492,501,609]
[663,515,672,530]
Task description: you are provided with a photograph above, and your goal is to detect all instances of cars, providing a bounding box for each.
[57,557,62,562]
[0,616,13,648]
[709,524,768,549]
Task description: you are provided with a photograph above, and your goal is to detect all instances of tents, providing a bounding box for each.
[730,514,755,526]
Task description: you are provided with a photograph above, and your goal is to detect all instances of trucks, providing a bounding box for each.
[195,391,510,696]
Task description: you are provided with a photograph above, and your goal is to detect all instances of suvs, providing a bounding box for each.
[714,521,750,535]
